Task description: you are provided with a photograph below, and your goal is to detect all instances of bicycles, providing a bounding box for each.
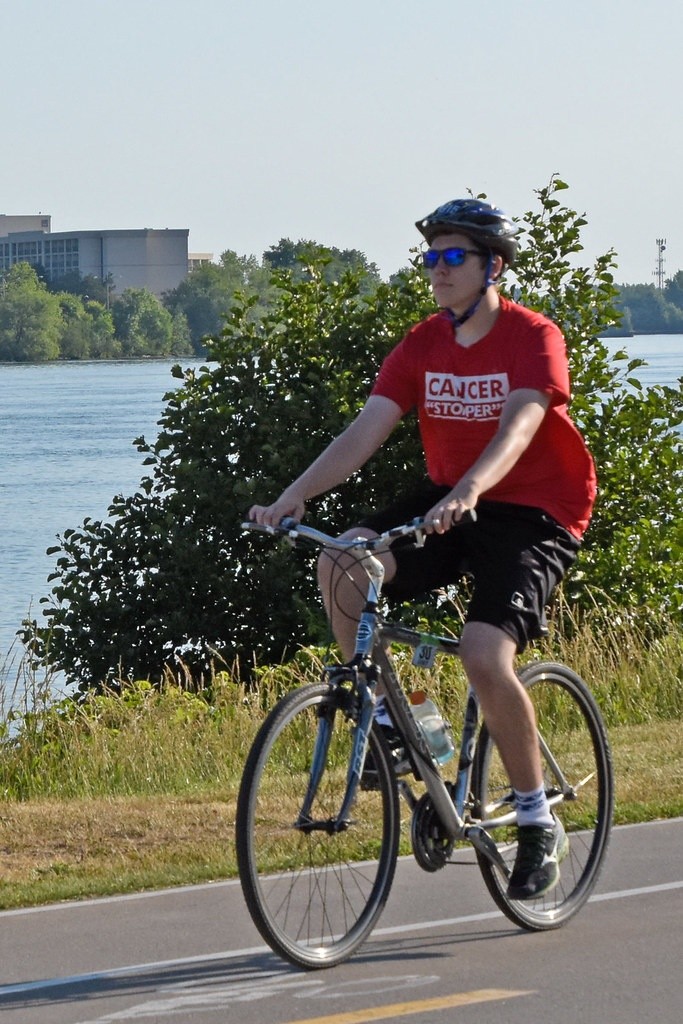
[235,509,615,971]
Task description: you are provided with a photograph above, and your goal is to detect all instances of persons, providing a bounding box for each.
[248,197,599,901]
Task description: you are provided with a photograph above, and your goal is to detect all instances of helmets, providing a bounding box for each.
[416,199,520,265]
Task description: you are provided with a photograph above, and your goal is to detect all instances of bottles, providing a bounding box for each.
[410,690,454,765]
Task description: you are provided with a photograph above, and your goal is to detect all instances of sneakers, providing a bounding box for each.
[362,724,408,774]
[506,809,568,900]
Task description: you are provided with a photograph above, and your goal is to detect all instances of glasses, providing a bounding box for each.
[423,248,482,271]
